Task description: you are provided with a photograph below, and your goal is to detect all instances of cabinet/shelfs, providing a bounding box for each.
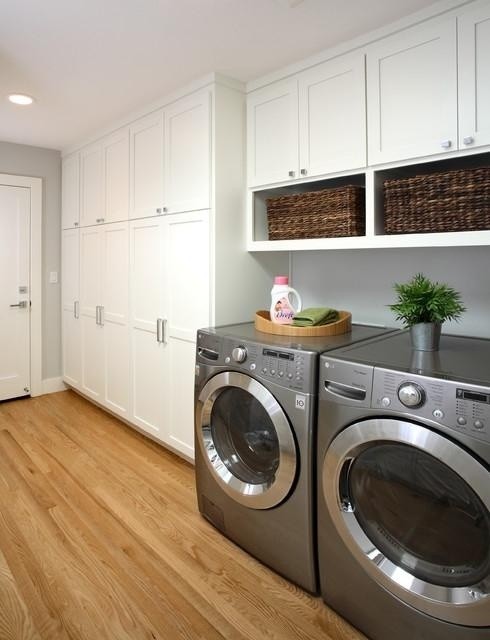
[61,153,82,230]
[82,83,243,227]
[245,49,370,247]
[129,215,269,462]
[61,230,79,393]
[368,1,490,242]
[82,223,130,418]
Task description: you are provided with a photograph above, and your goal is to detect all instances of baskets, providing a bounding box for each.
[265,184,365,241]
[383,166,490,234]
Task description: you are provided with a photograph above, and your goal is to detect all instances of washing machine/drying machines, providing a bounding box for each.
[194,320,402,596]
[317,328,490,640]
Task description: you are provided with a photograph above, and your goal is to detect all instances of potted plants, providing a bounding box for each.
[384,273,468,354]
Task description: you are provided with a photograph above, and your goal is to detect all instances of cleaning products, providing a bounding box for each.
[270,276,302,324]
